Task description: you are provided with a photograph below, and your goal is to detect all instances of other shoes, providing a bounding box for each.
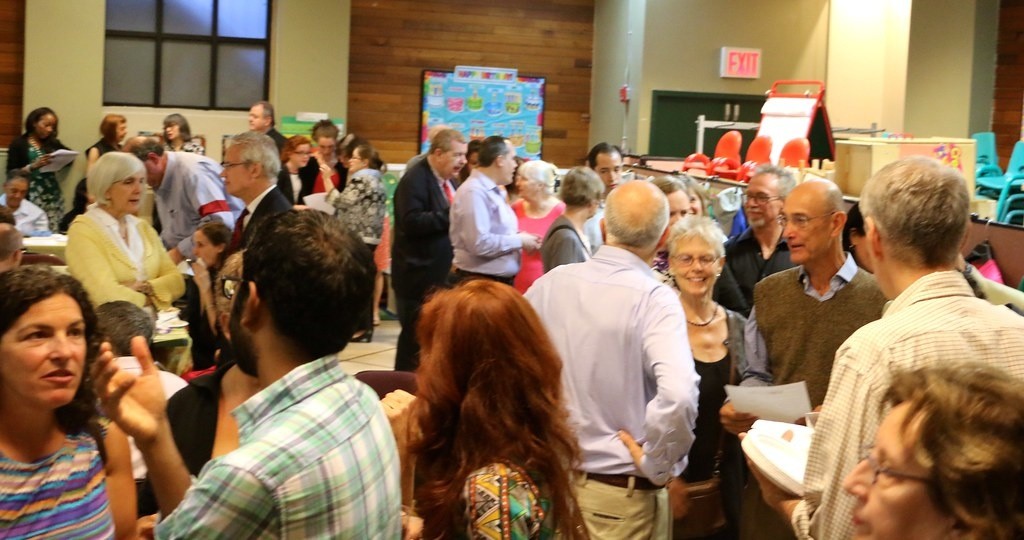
[372,317,380,325]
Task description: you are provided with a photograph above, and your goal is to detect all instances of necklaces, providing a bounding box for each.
[686,301,719,327]
[31,135,42,157]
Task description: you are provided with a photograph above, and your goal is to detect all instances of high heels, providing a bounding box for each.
[350,326,371,343]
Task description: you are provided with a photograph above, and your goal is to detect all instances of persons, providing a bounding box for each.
[0,207,402,540]
[248,101,289,153]
[720,179,888,540]
[85,114,127,204]
[65,150,194,377]
[843,353,1024,540]
[542,141,625,275]
[338,133,368,169]
[319,144,388,342]
[519,180,701,540]
[391,124,566,371]
[276,133,314,212]
[399,279,594,540]
[723,163,798,311]
[209,129,296,369]
[163,114,205,156]
[122,135,246,373]
[6,107,74,233]
[310,118,348,193]
[667,214,748,540]
[738,155,1024,540]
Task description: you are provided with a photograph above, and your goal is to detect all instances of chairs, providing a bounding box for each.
[680,129,811,182]
[972,131,1024,225]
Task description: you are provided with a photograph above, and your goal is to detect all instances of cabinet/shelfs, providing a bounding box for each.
[836,137,975,214]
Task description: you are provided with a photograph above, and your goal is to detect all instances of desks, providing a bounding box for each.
[26,233,192,378]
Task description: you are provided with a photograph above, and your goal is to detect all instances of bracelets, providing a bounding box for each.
[139,281,151,295]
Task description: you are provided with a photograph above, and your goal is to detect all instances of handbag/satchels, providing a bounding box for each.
[668,477,727,540]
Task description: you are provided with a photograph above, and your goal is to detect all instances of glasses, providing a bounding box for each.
[740,191,783,205]
[293,150,312,159]
[220,161,249,172]
[858,438,935,492]
[670,252,721,268]
[777,210,836,229]
[111,177,145,188]
[221,276,248,300]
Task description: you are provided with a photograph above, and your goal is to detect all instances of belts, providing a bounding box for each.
[457,269,515,282]
[581,471,652,490]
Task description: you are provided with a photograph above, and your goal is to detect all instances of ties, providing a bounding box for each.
[443,182,455,206]
